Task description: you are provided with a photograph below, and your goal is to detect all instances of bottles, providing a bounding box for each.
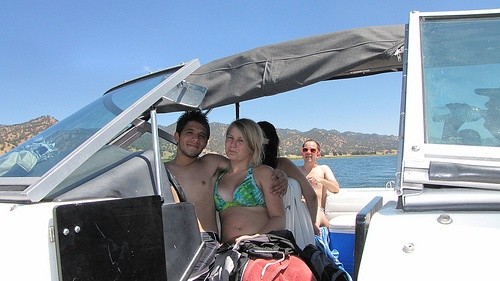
[431,106,486,122]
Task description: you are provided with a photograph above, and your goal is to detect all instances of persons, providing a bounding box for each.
[295,139,340,235]
[203,117,321,281]
[255,121,321,250]
[439,87,500,146]
[164,108,289,281]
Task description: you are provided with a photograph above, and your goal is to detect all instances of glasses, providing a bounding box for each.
[302,147,318,154]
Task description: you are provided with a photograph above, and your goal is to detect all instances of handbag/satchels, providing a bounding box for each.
[205,230,349,281]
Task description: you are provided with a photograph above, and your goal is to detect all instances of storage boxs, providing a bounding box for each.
[328,215,356,276]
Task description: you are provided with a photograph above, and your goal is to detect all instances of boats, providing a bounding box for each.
[0,9,500,281]
